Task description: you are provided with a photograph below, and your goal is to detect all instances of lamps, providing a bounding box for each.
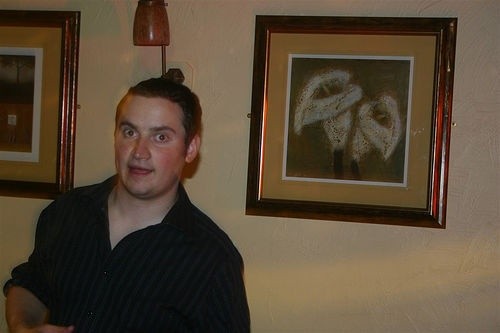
[132,1,186,86]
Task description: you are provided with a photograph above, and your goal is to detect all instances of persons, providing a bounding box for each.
[2,77,251,333]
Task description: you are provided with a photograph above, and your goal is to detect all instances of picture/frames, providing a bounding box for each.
[245,14,459,229]
[0,9,82,200]
